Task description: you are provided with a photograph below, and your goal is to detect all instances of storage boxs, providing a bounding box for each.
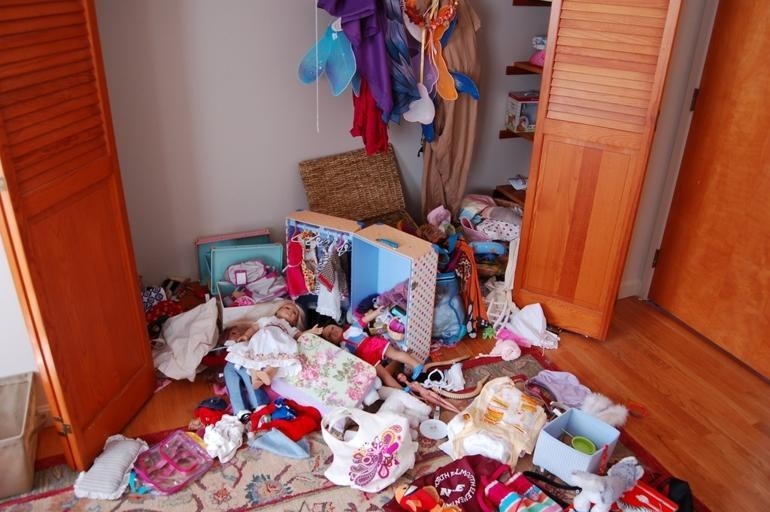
[189,227,284,293]
[1,372,40,499]
[503,90,542,134]
[210,243,286,328]
[530,409,622,476]
[281,207,358,319]
[348,225,438,356]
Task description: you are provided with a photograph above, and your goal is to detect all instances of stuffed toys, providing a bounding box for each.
[569,455,645,512]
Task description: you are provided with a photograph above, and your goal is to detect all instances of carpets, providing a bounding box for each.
[0,348,687,512]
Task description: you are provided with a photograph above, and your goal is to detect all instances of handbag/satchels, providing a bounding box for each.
[317,403,415,494]
[134,428,216,499]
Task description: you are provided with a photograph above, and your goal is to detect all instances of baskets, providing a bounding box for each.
[301,144,424,242]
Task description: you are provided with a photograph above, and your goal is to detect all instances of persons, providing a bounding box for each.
[321,306,425,394]
[237,300,304,391]
[392,370,460,415]
[222,326,269,424]
